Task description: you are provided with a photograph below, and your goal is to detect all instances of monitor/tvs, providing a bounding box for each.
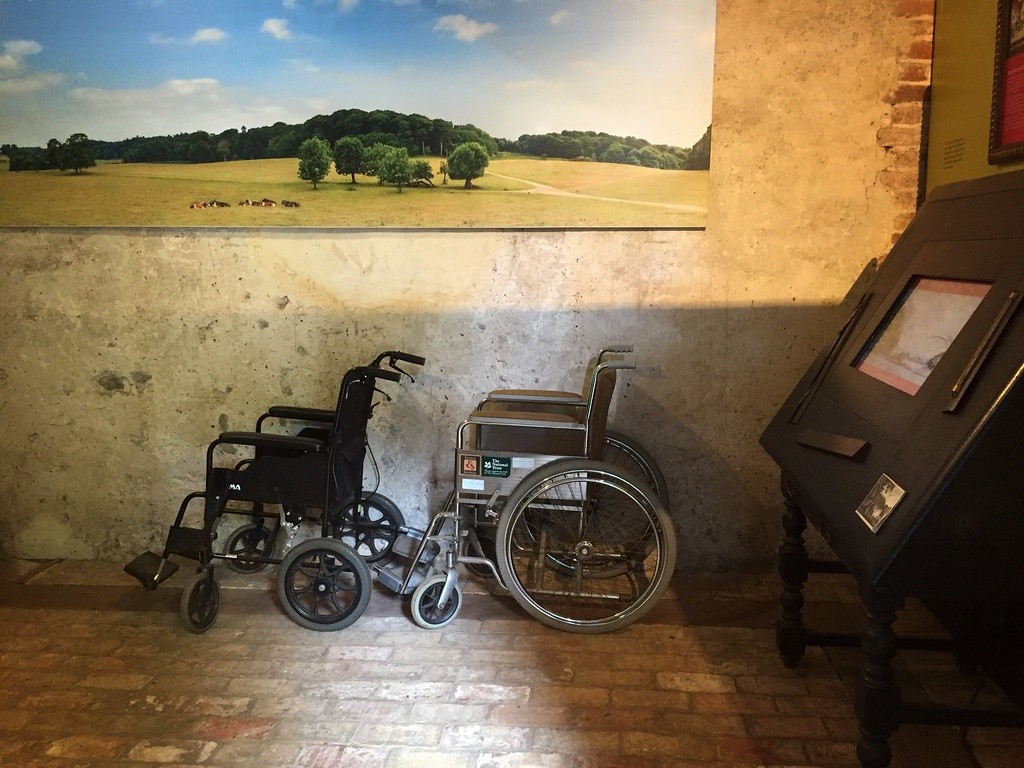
[851,273,997,397]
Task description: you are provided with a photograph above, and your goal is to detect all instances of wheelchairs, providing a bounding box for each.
[122,351,427,633]
[370,344,679,636]
